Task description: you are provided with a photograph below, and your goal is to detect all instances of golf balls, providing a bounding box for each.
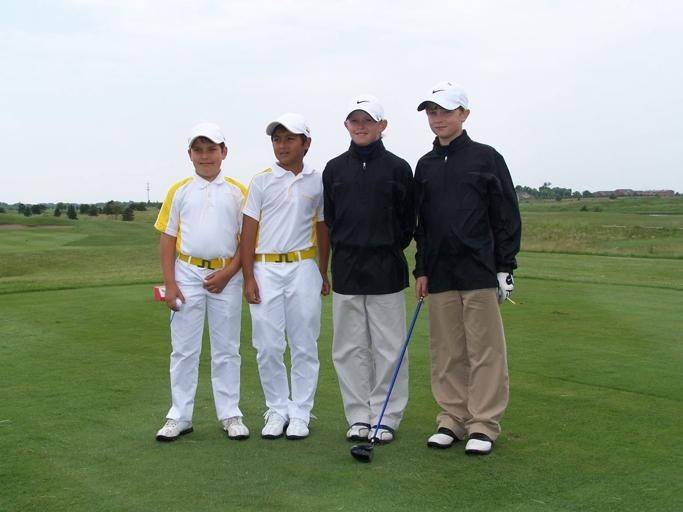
[176,298,182,308]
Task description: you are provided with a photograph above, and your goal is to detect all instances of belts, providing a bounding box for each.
[178,254,237,270]
[253,248,316,264]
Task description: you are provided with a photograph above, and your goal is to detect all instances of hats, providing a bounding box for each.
[416,81,470,112]
[187,123,226,155]
[265,113,314,141]
[345,96,384,125]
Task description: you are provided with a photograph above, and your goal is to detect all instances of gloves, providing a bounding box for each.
[496,269,517,305]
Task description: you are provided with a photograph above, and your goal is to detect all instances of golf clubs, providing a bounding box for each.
[350,294,424,464]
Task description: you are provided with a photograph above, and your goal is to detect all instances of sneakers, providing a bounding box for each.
[261,411,286,439]
[426,424,458,448]
[155,417,194,442]
[346,422,368,442]
[465,431,494,455]
[367,423,396,443]
[222,415,251,440]
[285,415,310,439]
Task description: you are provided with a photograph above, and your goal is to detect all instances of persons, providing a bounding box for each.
[412,81,522,454]
[241,115,332,440]
[153,126,251,443]
[323,96,416,446]
